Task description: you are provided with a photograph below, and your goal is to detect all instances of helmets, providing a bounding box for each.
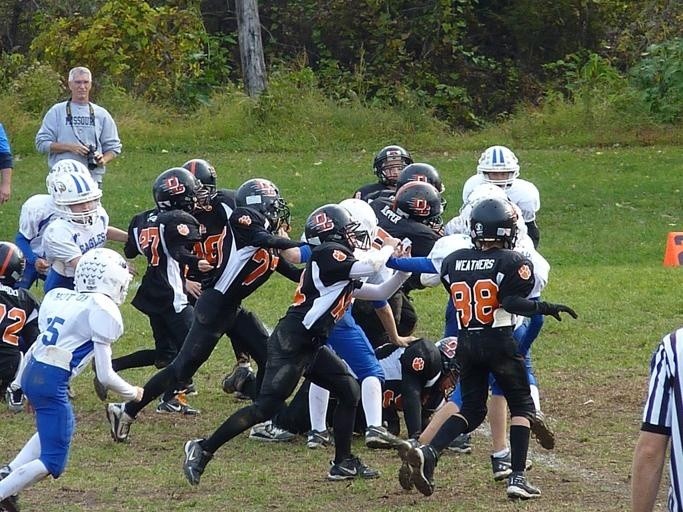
[74,247,131,306]
[47,172,100,206]
[181,158,217,201]
[45,159,91,194]
[302,145,521,259]
[234,178,291,234]
[151,167,213,214]
[0,240,26,288]
[434,335,461,402]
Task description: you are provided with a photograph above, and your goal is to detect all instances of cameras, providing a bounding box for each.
[87,144,97,169]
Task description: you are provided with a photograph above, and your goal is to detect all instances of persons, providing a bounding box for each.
[1,247,143,512]
[35,66,122,190]
[630,326,683,511]
[0,241,39,413]
[0,121,14,205]
[91,159,312,444]
[249,144,579,496]
[16,158,93,288]
[42,172,140,296]
[183,203,412,488]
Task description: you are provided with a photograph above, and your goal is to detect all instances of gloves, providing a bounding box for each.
[541,300,578,321]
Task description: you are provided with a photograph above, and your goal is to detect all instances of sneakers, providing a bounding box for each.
[105,402,132,444]
[91,356,111,401]
[66,385,77,400]
[4,376,24,411]
[0,465,21,512]
